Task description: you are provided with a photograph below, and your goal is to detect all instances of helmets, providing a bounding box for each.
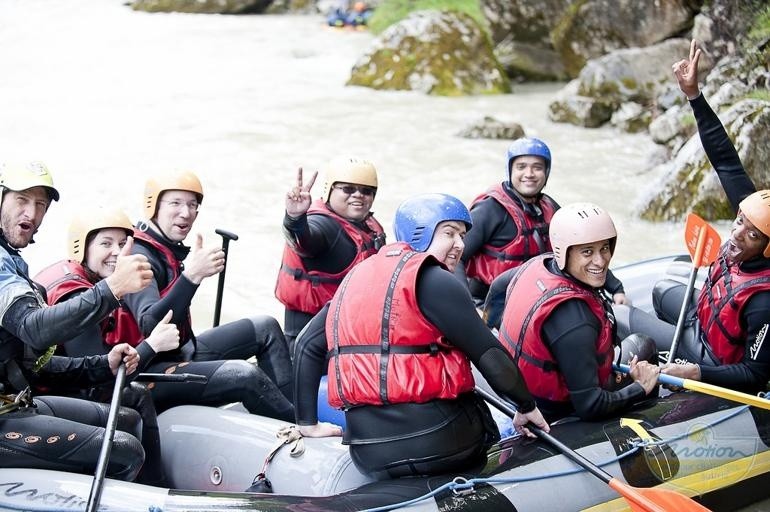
[143,166,203,222]
[322,152,379,206]
[549,202,618,273]
[2,158,59,202]
[68,201,134,266]
[505,136,552,188]
[737,189,770,260]
[394,191,473,253]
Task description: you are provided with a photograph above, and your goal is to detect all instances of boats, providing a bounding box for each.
[0,251,768,512]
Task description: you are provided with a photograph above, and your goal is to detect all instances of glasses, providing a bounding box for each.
[331,184,377,197]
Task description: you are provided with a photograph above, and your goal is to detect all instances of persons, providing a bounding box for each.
[0,155,155,484]
[30,200,181,489]
[290,191,552,483]
[611,37,770,394]
[483,203,661,422]
[274,155,388,361]
[453,137,629,327]
[120,166,294,424]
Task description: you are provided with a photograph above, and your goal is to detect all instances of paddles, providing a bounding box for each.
[609,360,766,411]
[475,384,713,512]
[666,212,721,363]
